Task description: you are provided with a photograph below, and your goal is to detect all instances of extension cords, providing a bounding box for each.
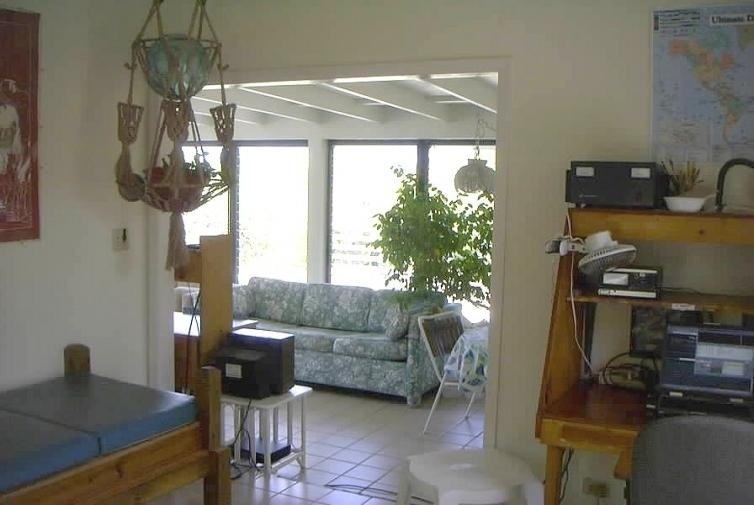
[597,372,649,391]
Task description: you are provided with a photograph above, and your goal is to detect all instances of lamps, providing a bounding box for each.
[117,0,240,270]
[456,113,495,194]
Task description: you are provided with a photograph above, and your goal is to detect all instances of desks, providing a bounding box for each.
[220,386,312,477]
[536,376,655,502]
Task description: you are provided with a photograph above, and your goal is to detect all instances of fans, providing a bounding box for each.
[543,231,638,277]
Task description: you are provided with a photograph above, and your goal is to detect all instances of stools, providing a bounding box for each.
[395,447,543,505]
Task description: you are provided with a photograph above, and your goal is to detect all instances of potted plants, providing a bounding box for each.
[658,158,706,213]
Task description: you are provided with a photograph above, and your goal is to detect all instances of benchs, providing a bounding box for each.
[0,343,235,505]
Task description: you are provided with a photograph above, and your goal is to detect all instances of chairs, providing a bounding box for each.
[621,414,752,503]
[416,311,484,434]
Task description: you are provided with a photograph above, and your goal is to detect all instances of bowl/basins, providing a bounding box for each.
[661,195,710,214]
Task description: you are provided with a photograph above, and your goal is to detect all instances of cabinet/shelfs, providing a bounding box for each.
[533,208,752,414]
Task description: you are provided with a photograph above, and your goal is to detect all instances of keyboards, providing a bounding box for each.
[657,397,754,423]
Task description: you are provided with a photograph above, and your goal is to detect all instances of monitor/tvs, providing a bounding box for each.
[659,321,754,404]
[215,327,295,400]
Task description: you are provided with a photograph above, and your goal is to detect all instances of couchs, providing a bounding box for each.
[173,278,446,405]
